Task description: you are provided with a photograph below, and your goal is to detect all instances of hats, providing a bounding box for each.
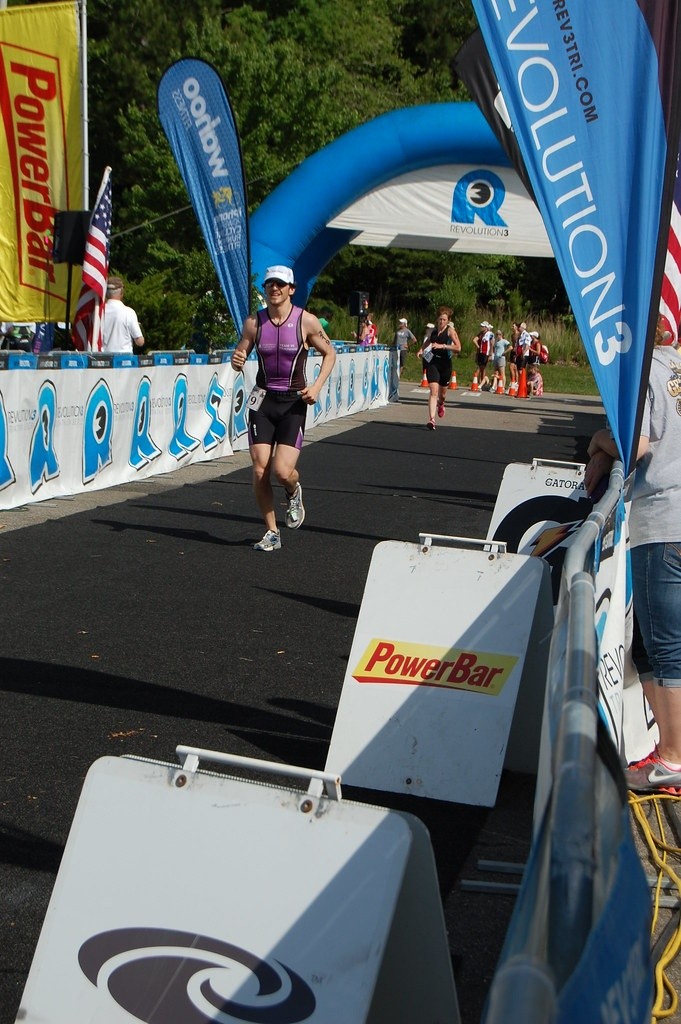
[399,318,407,324]
[424,322,435,328]
[480,321,489,326]
[107,277,124,290]
[516,322,527,329]
[264,266,293,283]
[528,331,539,338]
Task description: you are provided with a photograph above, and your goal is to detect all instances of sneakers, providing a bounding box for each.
[427,417,435,430]
[437,400,444,417]
[623,740,681,794]
[253,529,281,552]
[284,482,305,529]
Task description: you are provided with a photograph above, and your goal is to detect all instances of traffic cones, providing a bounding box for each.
[468,372,480,391]
[418,369,429,387]
[508,377,516,396]
[447,372,459,389]
[493,375,504,395]
[515,368,530,398]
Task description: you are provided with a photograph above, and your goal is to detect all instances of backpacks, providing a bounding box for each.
[534,341,548,364]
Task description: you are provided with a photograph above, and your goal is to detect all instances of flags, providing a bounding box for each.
[69,166,113,352]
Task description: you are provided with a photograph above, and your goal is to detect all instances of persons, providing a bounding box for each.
[230,265,336,551]
[350,306,545,431]
[101,277,145,353]
[585,312,681,795]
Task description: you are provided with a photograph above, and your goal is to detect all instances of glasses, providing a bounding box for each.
[265,280,289,287]
